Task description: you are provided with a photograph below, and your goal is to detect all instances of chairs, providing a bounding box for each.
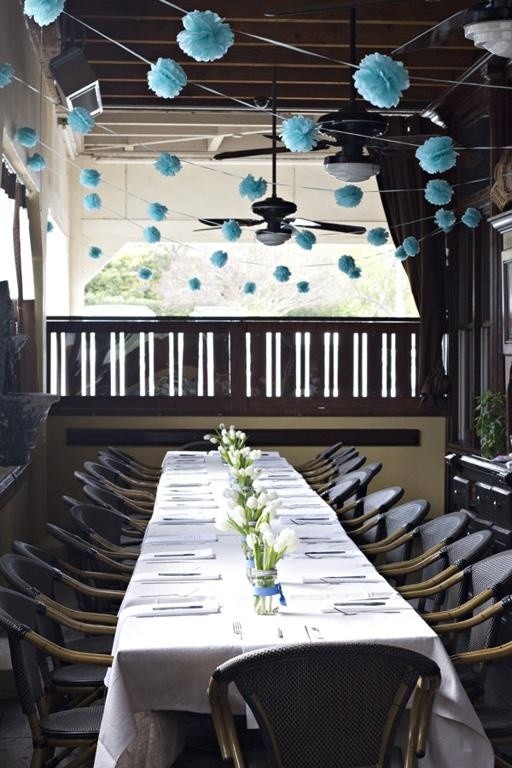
[179,437,218,450]
[2,443,162,679]
[208,643,442,763]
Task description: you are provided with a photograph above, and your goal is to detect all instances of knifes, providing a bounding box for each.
[334,602,386,607]
[155,553,195,558]
[305,550,346,555]
[158,572,201,577]
[321,575,366,580]
[152,605,202,610]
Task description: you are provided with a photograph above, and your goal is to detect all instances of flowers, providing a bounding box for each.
[202,422,248,448]
[216,494,281,553]
[248,523,298,612]
[196,69,367,232]
[224,487,281,521]
[219,445,262,470]
[231,467,261,487]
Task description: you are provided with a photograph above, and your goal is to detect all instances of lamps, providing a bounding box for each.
[255,230,292,245]
[49,48,102,119]
[463,21,512,59]
[322,155,380,184]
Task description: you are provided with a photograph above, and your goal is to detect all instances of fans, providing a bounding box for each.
[213,0,475,160]
[390,0,512,56]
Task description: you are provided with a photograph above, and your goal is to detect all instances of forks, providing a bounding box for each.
[306,553,354,559]
[291,519,330,526]
[334,607,402,618]
[233,621,247,655]
[321,578,381,584]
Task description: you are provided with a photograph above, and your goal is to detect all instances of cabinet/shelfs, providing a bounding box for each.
[445,455,511,560]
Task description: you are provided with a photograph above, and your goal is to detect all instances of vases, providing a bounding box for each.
[242,535,264,560]
[247,546,263,585]
[0,392,60,467]
[253,568,278,614]
